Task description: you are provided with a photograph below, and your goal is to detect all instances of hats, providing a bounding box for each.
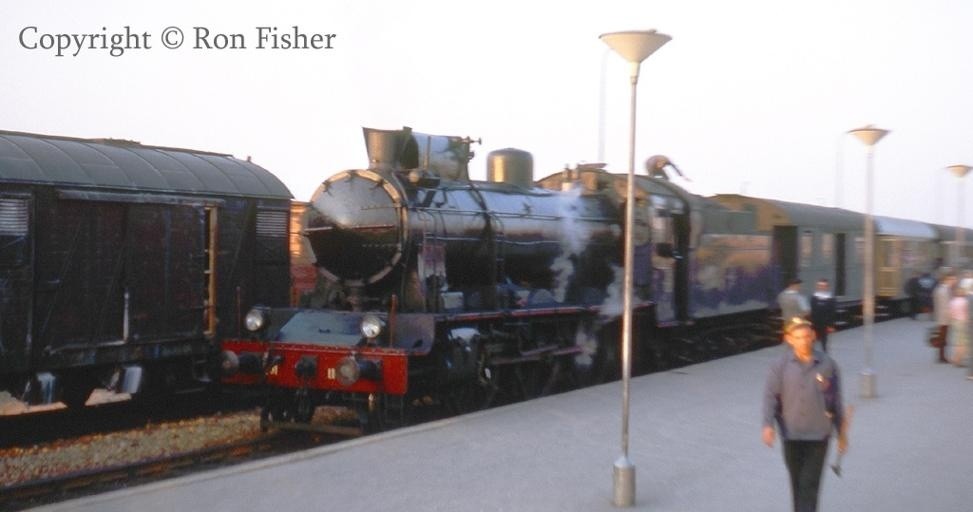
[783,315,815,333]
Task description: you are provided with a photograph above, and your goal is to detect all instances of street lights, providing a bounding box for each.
[596,29,672,509]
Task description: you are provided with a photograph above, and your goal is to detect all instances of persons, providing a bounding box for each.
[903,259,972,382]
[809,276,838,353]
[762,317,851,512]
[777,277,813,333]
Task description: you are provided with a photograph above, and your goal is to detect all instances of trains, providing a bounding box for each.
[1,126,973,432]
